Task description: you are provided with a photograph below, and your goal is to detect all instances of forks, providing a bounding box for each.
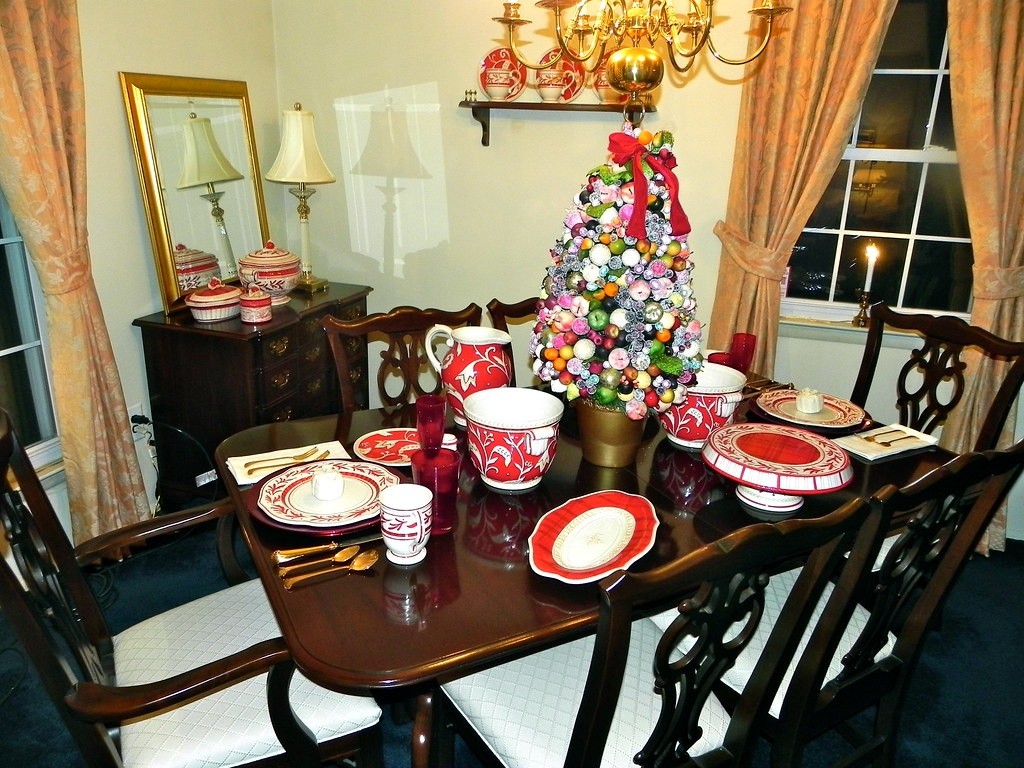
[855,430,919,448]
[244,447,319,468]
[247,450,330,476]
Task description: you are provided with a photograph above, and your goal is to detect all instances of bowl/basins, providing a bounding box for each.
[171,244,222,294]
[237,242,301,306]
[184,279,245,323]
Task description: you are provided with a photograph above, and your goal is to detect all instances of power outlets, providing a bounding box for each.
[128,402,148,443]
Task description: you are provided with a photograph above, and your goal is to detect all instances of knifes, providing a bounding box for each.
[271,532,384,568]
[743,383,794,400]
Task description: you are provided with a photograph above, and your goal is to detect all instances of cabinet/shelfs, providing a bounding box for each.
[133,281,372,510]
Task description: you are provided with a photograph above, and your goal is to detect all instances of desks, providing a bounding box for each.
[215,372,988,768]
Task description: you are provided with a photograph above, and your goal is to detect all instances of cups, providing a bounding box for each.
[486,68,521,102]
[411,448,458,535]
[417,396,446,459]
[239,293,272,323]
[385,567,438,631]
[708,332,756,375]
[461,489,560,563]
[424,535,461,608]
[378,483,434,565]
[649,440,712,519]
[538,69,575,104]
[597,69,630,104]
[463,387,565,491]
[657,362,751,454]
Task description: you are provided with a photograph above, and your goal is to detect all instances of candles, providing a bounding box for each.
[863,247,880,292]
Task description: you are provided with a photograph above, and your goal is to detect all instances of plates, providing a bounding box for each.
[528,489,659,585]
[478,46,529,102]
[245,459,413,538]
[525,573,604,614]
[352,428,457,467]
[591,46,636,103]
[534,46,588,104]
[747,389,873,436]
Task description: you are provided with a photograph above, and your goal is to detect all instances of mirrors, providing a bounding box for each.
[116,71,270,316]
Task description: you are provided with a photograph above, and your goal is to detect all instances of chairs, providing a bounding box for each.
[0,301,1024,767]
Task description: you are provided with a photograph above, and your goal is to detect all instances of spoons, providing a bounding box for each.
[278,545,360,578]
[283,549,380,590]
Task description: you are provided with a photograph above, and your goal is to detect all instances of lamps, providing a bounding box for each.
[173,112,245,277]
[264,104,337,293]
[490,0,794,128]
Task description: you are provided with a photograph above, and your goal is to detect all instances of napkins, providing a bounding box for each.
[225,440,352,486]
[830,422,941,461]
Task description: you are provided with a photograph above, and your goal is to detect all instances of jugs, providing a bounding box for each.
[424,322,512,427]
